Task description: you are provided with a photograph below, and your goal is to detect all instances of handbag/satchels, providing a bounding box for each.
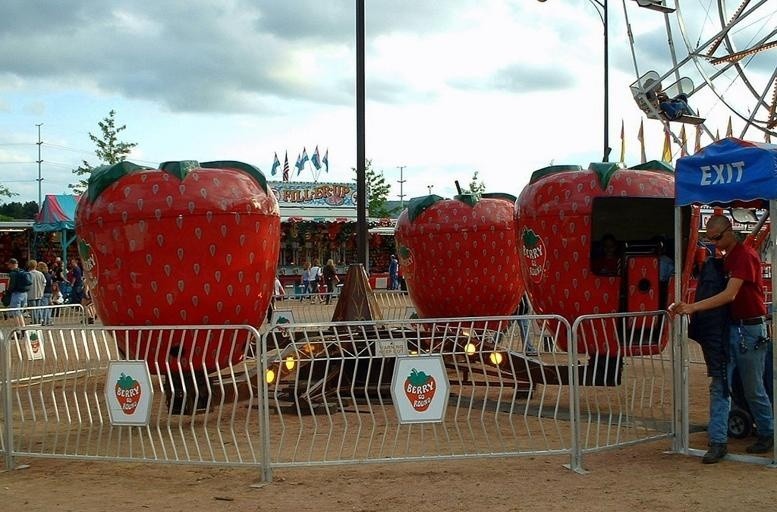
[315,268,323,281]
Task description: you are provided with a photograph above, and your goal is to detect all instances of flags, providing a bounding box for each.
[271,144,328,181]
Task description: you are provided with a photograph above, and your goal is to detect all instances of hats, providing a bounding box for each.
[5,258,18,265]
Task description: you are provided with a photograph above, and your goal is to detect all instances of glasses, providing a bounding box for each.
[706,224,732,241]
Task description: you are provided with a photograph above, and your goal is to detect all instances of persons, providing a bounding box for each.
[300,259,340,305]
[6,257,98,325]
[643,78,687,120]
[673,214,773,464]
[267,277,285,324]
[389,255,399,289]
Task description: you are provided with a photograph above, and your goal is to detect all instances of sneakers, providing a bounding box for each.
[700,445,728,465]
[80,315,97,325]
[745,433,773,456]
[10,329,26,340]
[300,297,335,306]
[24,312,61,326]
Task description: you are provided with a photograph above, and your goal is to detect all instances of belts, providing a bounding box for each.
[730,314,770,327]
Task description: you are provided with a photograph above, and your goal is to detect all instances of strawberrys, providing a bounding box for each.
[74,159,281,376]
[115,375,141,415]
[29,333,40,354]
[409,312,421,331]
[394,192,525,334]
[404,372,436,412]
[514,160,675,357]
[276,316,290,333]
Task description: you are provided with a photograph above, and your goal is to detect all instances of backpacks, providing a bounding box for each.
[17,269,33,292]
